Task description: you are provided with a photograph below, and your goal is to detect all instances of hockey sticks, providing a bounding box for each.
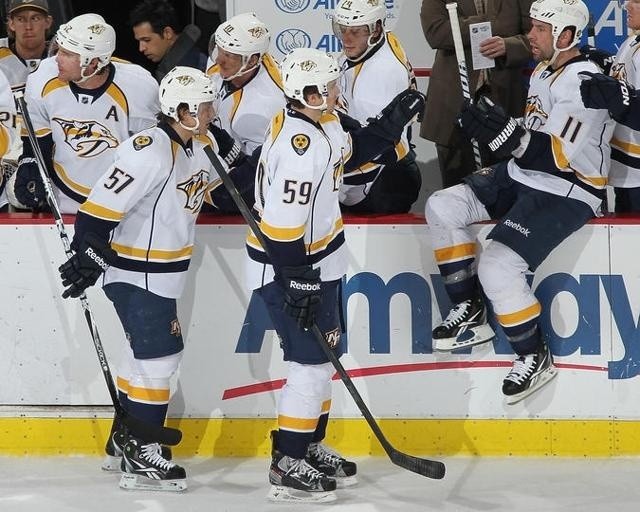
[203,144,446,479]
[14,90,182,446]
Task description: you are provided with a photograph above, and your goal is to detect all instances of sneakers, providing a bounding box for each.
[432,294,487,339]
[106,427,186,480]
[503,343,553,395]
[269,442,356,492]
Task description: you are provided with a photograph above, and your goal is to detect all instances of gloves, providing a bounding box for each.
[282,265,323,327]
[14,155,45,208]
[368,90,427,143]
[59,233,116,298]
[578,71,640,133]
[457,104,526,156]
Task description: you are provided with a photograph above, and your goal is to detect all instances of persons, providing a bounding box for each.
[247,47,428,492]
[57,66,263,480]
[424,1,616,396]
[0,0,639,214]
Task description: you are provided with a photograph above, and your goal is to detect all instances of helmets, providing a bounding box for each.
[159,0,386,131]
[55,13,116,78]
[529,0,590,51]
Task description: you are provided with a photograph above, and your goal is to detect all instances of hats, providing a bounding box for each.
[9,0,49,14]
